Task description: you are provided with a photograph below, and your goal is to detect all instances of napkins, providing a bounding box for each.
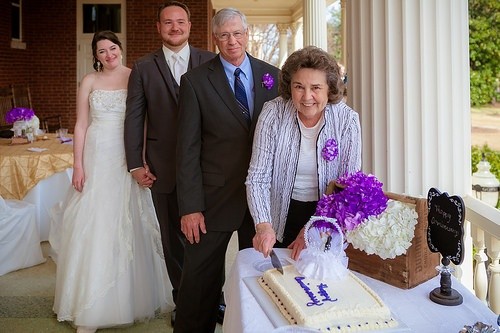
[27,147,49,153]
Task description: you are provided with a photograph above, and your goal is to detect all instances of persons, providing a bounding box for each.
[50,31,174,333]
[173,9,282,333]
[246,45,361,261]
[124,0,226,323]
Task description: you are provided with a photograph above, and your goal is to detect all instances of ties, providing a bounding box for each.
[172,53,183,87]
[234,68,252,131]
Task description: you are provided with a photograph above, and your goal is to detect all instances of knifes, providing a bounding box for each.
[270,250,283,275]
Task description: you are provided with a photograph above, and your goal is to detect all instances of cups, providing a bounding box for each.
[59,128,68,139]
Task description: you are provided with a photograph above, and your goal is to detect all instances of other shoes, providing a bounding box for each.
[215,304,226,325]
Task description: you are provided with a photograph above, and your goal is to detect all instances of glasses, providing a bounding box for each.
[214,30,246,41]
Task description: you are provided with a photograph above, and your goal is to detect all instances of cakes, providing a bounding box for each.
[257,216,399,333]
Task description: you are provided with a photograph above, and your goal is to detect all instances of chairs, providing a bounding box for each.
[10,82,63,133]
[0,93,14,129]
[0,194,48,277]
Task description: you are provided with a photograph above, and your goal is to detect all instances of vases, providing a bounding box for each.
[12,115,42,137]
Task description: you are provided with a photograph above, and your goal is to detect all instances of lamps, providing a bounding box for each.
[472,149,500,207]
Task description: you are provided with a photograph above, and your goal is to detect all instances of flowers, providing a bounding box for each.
[262,72,275,91]
[5,105,35,125]
[313,170,419,261]
[322,136,340,161]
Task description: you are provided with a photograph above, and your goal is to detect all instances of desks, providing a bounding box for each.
[0,132,75,244]
[217,246,500,333]
